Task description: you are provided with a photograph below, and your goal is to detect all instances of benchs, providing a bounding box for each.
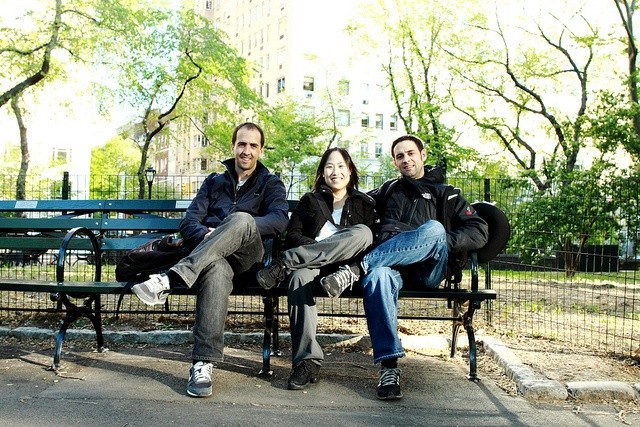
[1,199,497,380]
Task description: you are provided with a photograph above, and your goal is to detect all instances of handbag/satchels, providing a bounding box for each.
[314,192,344,231]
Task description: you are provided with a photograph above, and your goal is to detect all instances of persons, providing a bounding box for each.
[319,135,489,400]
[256,147,381,391]
[131,122,288,397]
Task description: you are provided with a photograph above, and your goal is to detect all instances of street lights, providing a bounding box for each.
[145,167,156,199]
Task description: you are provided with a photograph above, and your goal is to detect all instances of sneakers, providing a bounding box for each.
[187,361,214,397]
[375,368,403,399]
[130,272,171,307]
[320,264,361,300]
[255,258,285,290]
[288,360,321,390]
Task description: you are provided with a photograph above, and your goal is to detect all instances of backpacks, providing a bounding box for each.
[114,236,185,282]
[380,179,469,283]
